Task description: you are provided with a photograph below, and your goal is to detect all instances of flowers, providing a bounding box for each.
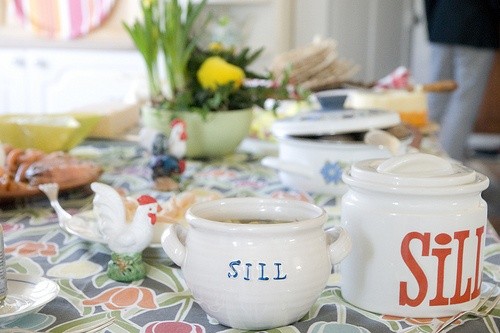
[122,1,324,145]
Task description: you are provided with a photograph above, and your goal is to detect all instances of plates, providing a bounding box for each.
[0,159,103,198]
[65,210,161,249]
[0,271,60,328]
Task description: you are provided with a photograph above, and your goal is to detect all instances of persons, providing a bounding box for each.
[419,0,500,156]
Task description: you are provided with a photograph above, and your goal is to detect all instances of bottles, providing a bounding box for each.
[0,224,8,301]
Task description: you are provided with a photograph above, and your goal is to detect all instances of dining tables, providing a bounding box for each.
[0,78,499,332]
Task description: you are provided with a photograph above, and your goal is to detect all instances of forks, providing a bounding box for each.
[432,281,500,333]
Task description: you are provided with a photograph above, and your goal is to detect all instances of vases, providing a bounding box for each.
[137,105,251,163]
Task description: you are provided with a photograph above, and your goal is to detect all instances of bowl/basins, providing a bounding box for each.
[269,94,416,195]
[1,113,103,154]
[339,152,490,319]
[161,198,354,331]
